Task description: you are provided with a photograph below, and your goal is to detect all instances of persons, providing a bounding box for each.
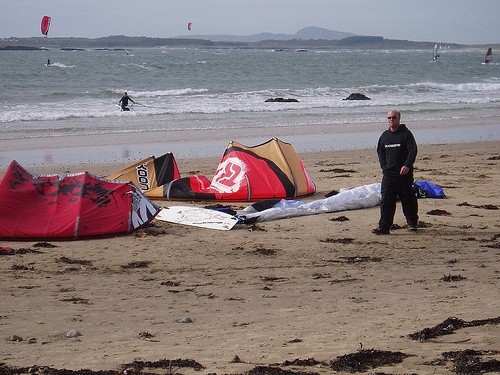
[47,59,51,66]
[371,109,419,235]
[119,92,136,112]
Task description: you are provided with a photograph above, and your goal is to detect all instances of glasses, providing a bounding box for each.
[387,116,399,119]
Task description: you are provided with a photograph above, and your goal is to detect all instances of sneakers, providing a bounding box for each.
[372,227,390,234]
[409,225,417,230]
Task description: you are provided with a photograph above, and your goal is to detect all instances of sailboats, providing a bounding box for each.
[481,48,496,64]
[432,43,443,61]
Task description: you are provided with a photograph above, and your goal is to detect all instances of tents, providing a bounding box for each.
[106,137,317,202]
[0,160,163,241]
